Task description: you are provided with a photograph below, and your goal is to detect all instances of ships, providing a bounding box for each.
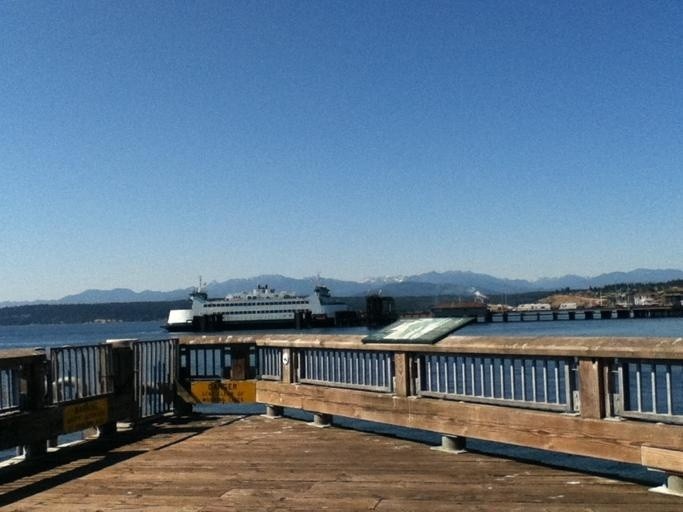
[160,283,357,332]
